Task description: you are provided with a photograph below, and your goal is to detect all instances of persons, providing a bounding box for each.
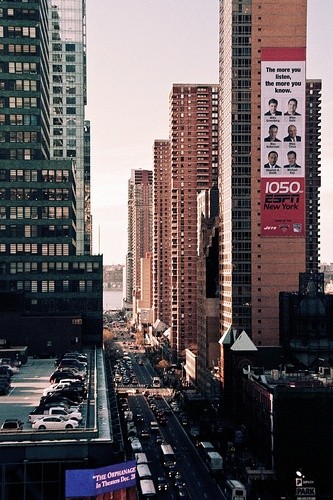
[263,151,281,168]
[263,125,283,142]
[284,124,301,142]
[284,97,302,116]
[264,99,283,116]
[283,151,301,168]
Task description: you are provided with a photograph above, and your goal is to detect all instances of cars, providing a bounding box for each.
[106,330,249,500]
[0,348,90,433]
